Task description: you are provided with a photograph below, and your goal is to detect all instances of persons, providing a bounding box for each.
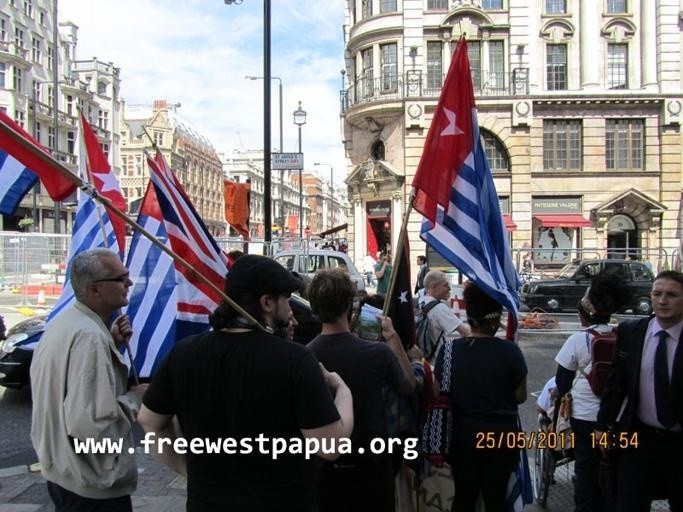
[232,240,682,512]
[135,255,355,511]
[27,250,151,512]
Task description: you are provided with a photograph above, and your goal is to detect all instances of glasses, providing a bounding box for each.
[94,272,129,283]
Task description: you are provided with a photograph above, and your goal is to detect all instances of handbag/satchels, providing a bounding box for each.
[414,391,457,469]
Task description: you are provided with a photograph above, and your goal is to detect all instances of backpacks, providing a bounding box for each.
[575,327,621,398]
[414,297,445,364]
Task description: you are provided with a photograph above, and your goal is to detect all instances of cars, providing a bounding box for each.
[0,282,329,391]
[274,249,365,295]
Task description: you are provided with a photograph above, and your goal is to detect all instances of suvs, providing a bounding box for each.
[522,259,656,316]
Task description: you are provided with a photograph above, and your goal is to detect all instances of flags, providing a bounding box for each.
[408,42,521,339]
[18,115,127,351]
[1,110,74,217]
[118,147,227,378]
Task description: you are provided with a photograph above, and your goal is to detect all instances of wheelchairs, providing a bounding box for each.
[534,395,575,505]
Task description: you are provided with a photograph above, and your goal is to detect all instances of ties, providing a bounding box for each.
[653,330,677,430]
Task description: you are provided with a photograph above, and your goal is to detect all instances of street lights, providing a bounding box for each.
[314,162,335,243]
[292,99,306,238]
[30,77,66,234]
[247,75,285,239]
[225,0,274,252]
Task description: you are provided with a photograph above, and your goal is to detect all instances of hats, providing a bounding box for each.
[223,255,305,295]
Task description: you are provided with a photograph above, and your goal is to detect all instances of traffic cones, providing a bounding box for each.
[36,283,49,306]
[452,295,461,318]
